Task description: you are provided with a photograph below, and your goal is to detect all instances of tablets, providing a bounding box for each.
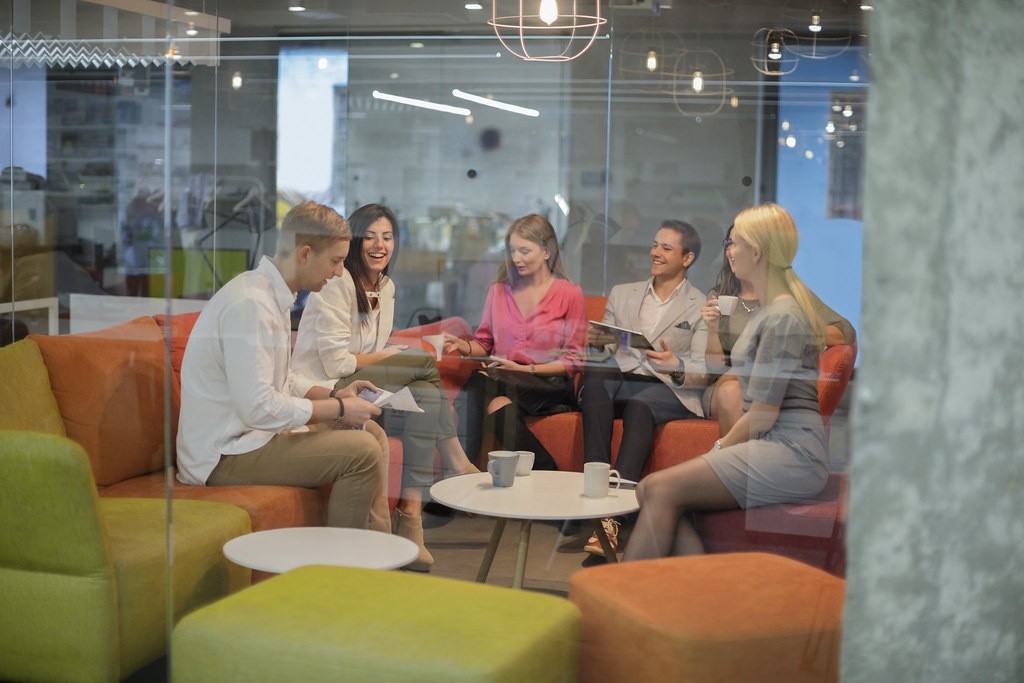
[588,321,656,353]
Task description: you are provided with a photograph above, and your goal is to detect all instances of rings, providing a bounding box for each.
[707,312,709,316]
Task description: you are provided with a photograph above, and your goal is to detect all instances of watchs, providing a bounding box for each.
[672,358,685,378]
[714,439,721,449]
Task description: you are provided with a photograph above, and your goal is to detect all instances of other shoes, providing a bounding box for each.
[583,518,621,557]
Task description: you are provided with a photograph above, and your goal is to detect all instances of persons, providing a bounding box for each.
[424,213,586,534]
[174,202,392,534]
[291,204,482,572]
[124,188,181,298]
[575,219,709,558]
[623,203,830,562]
[702,224,856,436]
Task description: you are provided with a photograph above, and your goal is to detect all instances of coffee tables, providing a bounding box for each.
[431,472,641,594]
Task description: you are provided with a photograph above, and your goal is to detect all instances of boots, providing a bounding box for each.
[392,507,434,572]
[443,463,482,479]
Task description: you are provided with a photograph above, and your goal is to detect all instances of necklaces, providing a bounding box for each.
[741,299,754,312]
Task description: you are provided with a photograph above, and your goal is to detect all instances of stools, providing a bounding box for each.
[171,563,581,683]
[700,471,850,580]
[574,553,845,683]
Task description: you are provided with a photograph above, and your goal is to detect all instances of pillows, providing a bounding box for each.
[154,315,201,384]
[0,340,65,438]
[27,317,181,486]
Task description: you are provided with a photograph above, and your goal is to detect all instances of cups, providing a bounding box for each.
[515,451,535,476]
[715,295,739,316]
[424,334,445,362]
[584,462,620,498]
[487,450,519,487]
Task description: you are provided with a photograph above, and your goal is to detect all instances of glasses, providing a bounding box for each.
[722,238,733,250]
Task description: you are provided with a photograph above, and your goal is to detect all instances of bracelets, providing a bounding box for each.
[466,341,472,355]
[530,364,536,375]
[330,389,338,397]
[336,397,345,424]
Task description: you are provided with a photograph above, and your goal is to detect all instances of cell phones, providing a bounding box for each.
[609,482,638,491]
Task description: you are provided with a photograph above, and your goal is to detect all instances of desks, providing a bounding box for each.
[226,526,417,573]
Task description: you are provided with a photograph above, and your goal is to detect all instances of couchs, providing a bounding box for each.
[0,313,473,683]
[477,297,854,479]
